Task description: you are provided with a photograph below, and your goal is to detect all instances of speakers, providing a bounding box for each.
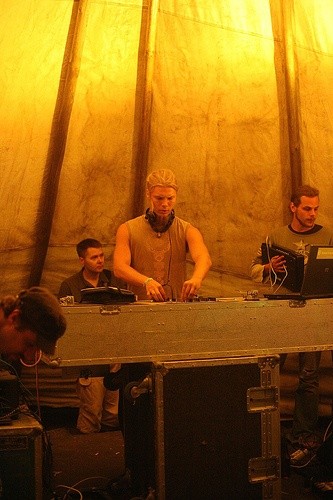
[44,430,126,493]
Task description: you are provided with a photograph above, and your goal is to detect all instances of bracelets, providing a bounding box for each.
[143,278,154,286]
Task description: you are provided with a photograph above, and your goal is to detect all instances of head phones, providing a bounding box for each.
[145,207,175,233]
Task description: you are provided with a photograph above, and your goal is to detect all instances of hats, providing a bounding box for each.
[16,287,66,356]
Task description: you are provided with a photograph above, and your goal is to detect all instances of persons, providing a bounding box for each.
[247,183,333,448]
[59,239,129,434]
[112,168,214,439]
[0,285,67,361]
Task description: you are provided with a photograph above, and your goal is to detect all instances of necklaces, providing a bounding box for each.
[146,208,176,238]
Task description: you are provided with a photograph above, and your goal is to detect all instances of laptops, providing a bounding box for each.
[263,245,333,300]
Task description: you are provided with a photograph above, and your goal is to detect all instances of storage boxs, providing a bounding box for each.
[150,354,282,500]
[0,413,42,500]
